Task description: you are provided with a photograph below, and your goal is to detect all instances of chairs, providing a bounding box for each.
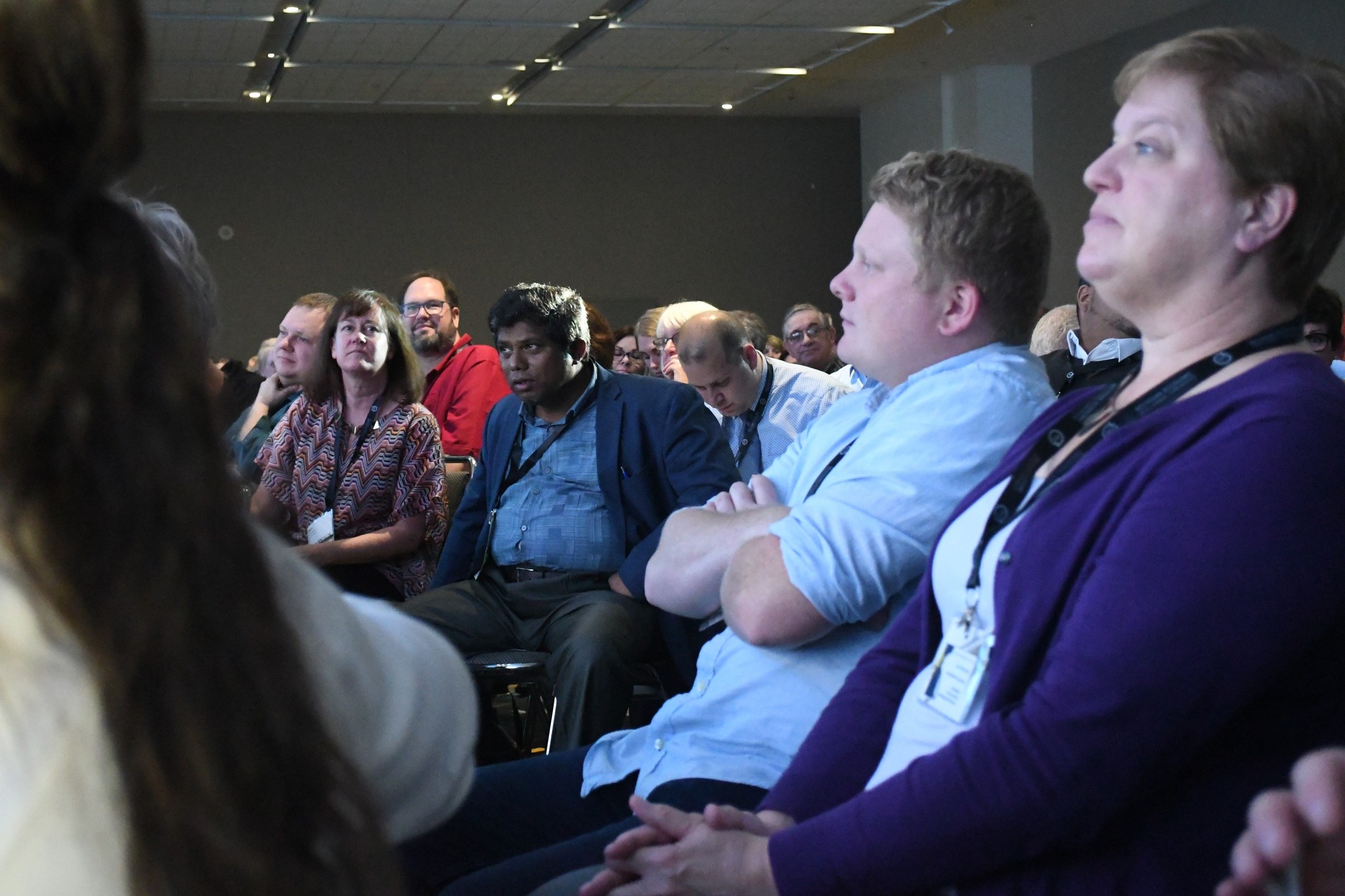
[443,455,478,525]
[463,649,551,759]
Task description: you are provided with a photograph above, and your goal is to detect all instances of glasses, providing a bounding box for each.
[652,331,679,350]
[613,349,643,365]
[400,299,449,317]
[785,326,826,341]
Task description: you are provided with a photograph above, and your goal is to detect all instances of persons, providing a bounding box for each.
[530,24,1345,896]
[210,271,868,766]
[0,0,480,896]
[388,145,1054,896]
[1029,268,1345,397]
[1215,750,1345,896]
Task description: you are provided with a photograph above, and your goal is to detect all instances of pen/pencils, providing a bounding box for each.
[621,467,627,478]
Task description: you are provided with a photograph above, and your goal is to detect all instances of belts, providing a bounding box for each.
[506,565,560,585]
